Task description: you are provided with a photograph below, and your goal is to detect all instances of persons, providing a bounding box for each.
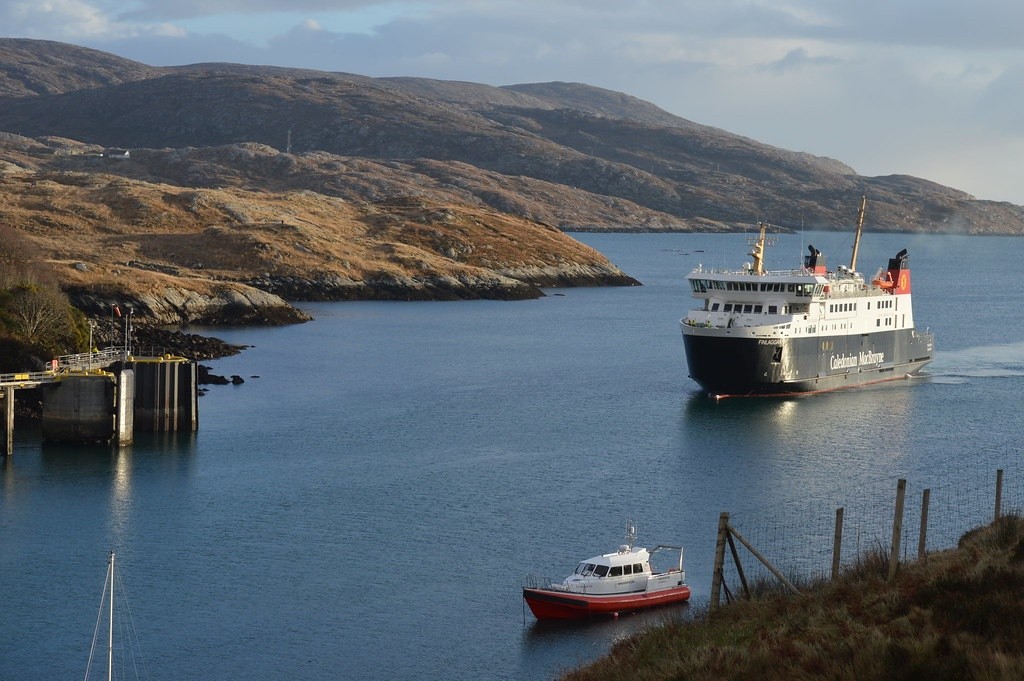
[706,321,712,328]
[689,320,695,326]
[61,350,69,371]
[93,345,98,363]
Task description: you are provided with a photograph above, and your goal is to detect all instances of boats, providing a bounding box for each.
[678,194,937,397]
[513,514,692,621]
